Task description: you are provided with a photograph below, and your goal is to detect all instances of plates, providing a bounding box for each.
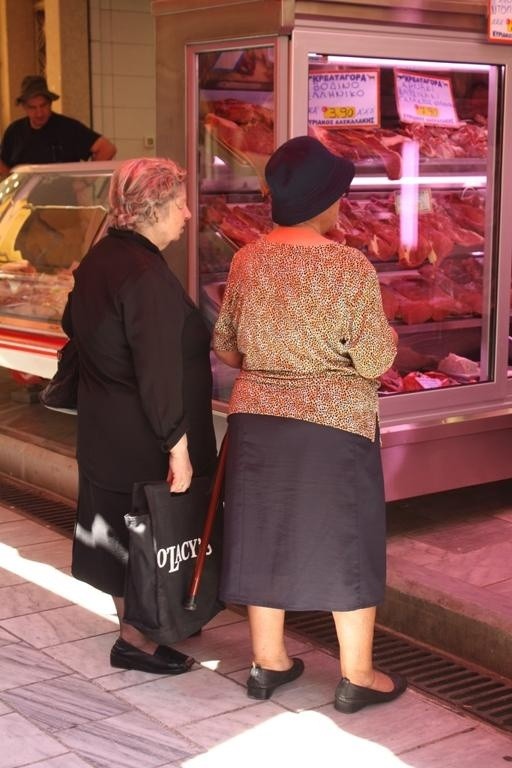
[439,368,480,382]
[375,388,396,397]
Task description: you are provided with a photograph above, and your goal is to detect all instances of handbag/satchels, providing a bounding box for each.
[39,339,80,416]
[120,477,228,651]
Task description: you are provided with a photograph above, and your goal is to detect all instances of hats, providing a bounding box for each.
[15,74,60,107]
[264,136,356,227]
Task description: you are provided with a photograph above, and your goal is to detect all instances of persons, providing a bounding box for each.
[206,135,411,715]
[1,73,118,182]
[60,157,223,675]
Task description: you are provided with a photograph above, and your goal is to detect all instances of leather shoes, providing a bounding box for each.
[333,670,408,715]
[110,634,195,674]
[244,657,305,702]
